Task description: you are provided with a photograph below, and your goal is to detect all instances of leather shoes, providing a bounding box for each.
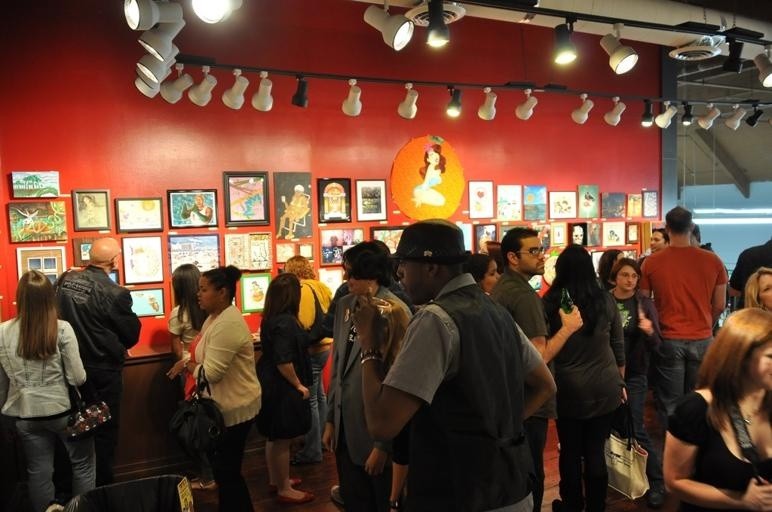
[268,478,305,490]
[272,493,316,504]
[188,476,216,490]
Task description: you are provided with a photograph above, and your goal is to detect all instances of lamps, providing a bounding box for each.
[681,104,694,126]
[425,0,450,48]
[723,41,744,74]
[697,103,721,130]
[221,69,250,110]
[552,18,578,66]
[640,101,654,127]
[570,94,594,125]
[514,89,538,121]
[723,105,746,131]
[446,87,463,118]
[654,101,678,129]
[744,105,764,128]
[341,79,362,117]
[753,44,772,88]
[291,76,309,108]
[600,23,639,76]
[397,83,418,119]
[251,72,274,112]
[138,18,187,63]
[603,97,626,126]
[135,68,173,99]
[160,64,195,105]
[124,1,184,32]
[136,43,181,84]
[188,66,218,108]
[363,0,415,51]
[477,87,498,121]
[191,0,243,24]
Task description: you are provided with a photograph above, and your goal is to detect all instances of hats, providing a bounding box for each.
[387,218,473,265]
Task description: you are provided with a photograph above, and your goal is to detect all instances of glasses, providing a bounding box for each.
[618,270,640,280]
[514,246,546,257]
[341,269,370,280]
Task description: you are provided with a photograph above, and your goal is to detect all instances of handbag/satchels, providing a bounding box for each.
[170,364,228,470]
[604,404,651,501]
[66,384,114,442]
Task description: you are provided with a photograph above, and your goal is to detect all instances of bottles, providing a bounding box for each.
[560,286,574,315]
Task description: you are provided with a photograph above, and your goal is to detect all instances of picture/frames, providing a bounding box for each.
[240,273,271,313]
[115,198,164,234]
[72,236,104,268]
[550,222,567,248]
[318,227,365,267]
[299,243,314,262]
[223,170,271,228]
[355,178,388,222]
[629,192,641,218]
[524,184,549,221]
[626,222,640,244]
[167,233,221,274]
[166,188,219,229]
[569,223,587,248]
[469,180,495,218]
[121,235,165,285]
[8,200,68,243]
[579,185,600,219]
[496,184,524,222]
[473,223,497,255]
[248,232,274,272]
[72,188,113,232]
[603,222,626,247]
[318,177,352,222]
[548,190,578,219]
[10,169,60,199]
[642,191,659,218]
[273,171,313,241]
[369,226,407,254]
[601,192,626,220]
[17,246,68,284]
[129,287,166,318]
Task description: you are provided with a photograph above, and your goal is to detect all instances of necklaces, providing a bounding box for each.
[740,410,758,427]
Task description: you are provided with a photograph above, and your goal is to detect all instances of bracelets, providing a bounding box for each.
[359,348,383,367]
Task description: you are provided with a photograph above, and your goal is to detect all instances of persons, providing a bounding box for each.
[153,265,263,511]
[351,217,556,512]
[748,260,772,317]
[688,221,715,255]
[250,272,320,503]
[607,259,670,507]
[542,244,630,512]
[320,250,368,508]
[53,238,142,489]
[0,271,99,512]
[318,247,414,511]
[659,306,772,512]
[283,255,339,467]
[489,226,583,512]
[725,230,772,309]
[640,206,730,426]
[169,265,218,419]
[638,224,669,259]
[461,248,501,298]
[599,248,623,290]
